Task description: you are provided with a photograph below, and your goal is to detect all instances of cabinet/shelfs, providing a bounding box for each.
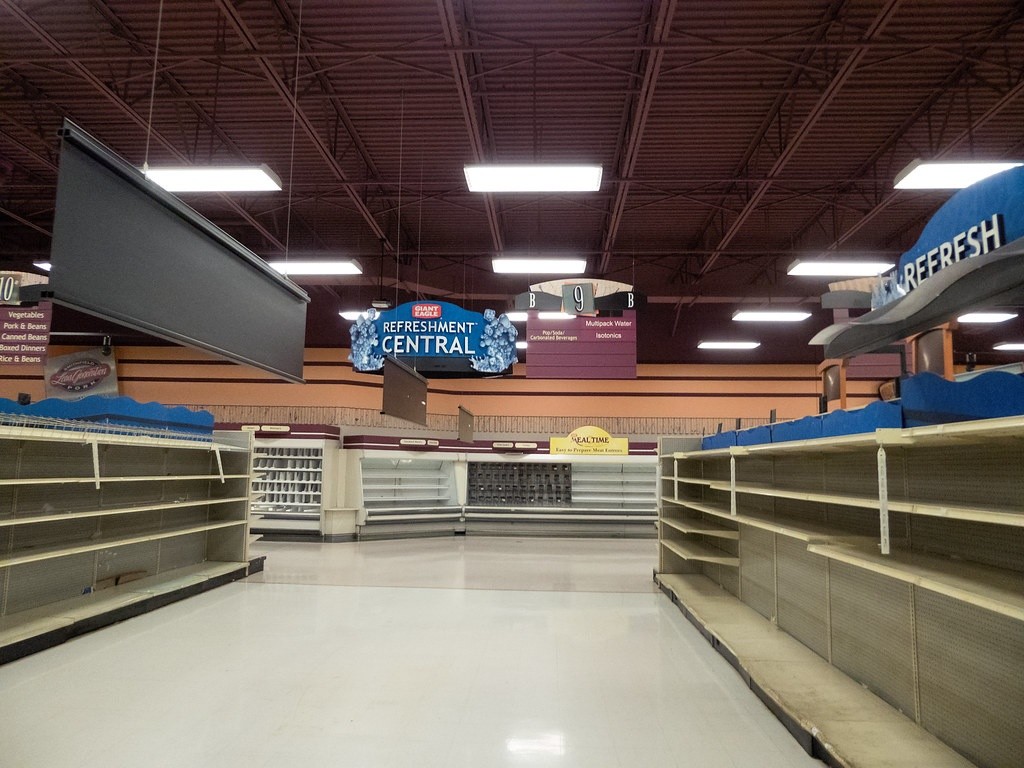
[213,421,659,543]
[0,412,267,667]
[653,414,1024,768]
